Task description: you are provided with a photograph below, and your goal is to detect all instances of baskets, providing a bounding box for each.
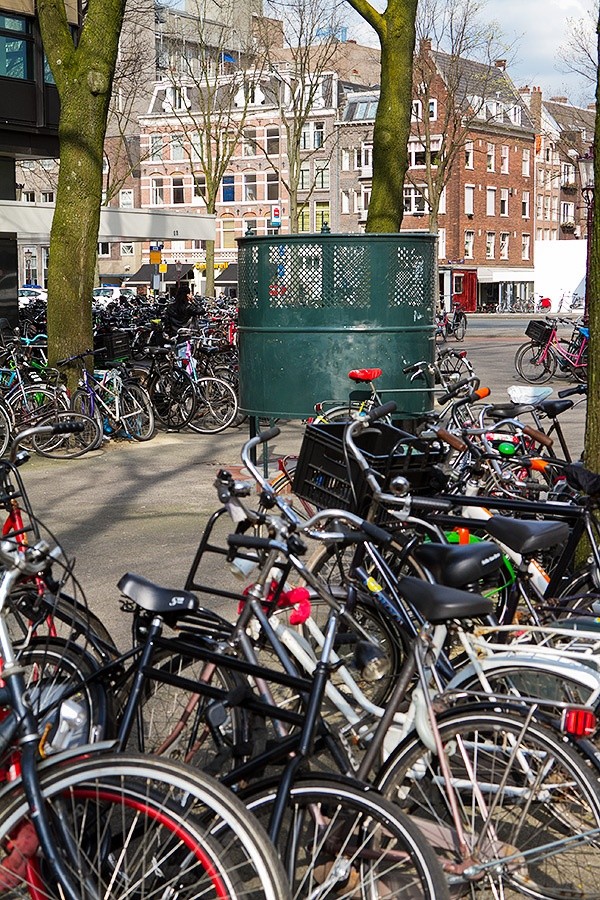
[294,421,441,527]
[523,320,553,343]
[93,331,133,364]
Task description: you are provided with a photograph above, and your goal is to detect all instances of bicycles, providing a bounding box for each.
[0,287,281,468]
[436,290,590,398]
[0,346,600,900]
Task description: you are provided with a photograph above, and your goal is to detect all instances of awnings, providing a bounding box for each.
[213,263,279,285]
[122,264,195,285]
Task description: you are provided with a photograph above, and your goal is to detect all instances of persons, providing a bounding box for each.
[196,292,200,295]
[219,292,225,299]
[163,284,206,342]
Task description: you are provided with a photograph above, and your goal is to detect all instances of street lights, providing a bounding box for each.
[176,260,183,287]
[24,248,33,286]
